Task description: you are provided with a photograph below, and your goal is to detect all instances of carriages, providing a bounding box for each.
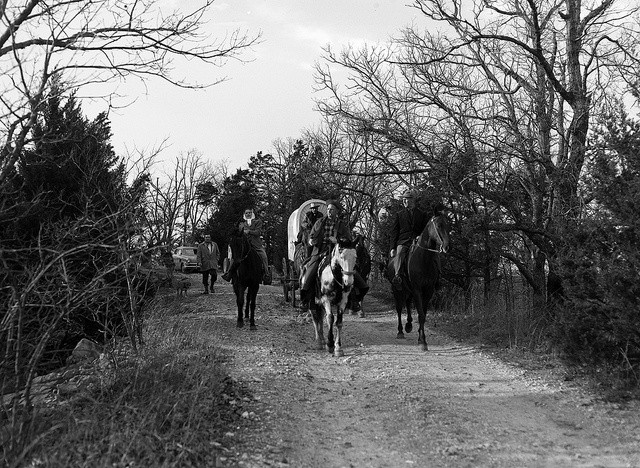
[273,198,371,318]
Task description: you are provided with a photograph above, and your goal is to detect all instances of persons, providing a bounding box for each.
[389,190,427,292]
[298,200,369,313]
[197,233,221,294]
[294,203,324,245]
[222,208,270,285]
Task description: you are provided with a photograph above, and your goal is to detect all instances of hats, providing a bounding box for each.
[326,199,342,210]
[309,202,320,208]
[398,190,414,198]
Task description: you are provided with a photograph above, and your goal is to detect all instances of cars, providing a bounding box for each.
[172,246,202,273]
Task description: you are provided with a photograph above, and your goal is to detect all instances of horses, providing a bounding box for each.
[293,224,314,311]
[308,234,361,357]
[388,205,452,352]
[230,224,263,331]
